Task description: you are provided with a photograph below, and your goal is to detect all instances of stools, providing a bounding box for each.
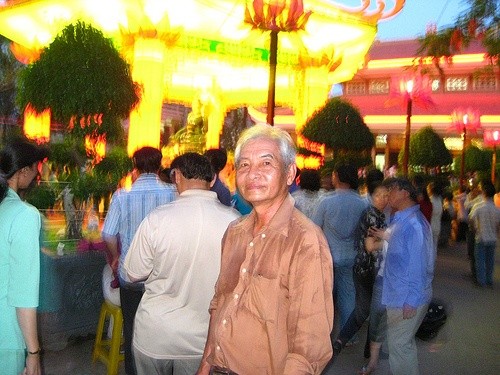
[92,299,125,375]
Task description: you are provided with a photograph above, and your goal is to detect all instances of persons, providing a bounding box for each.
[196,124,334,375]
[102,147,500,375]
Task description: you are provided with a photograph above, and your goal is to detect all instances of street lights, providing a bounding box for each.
[388,71,432,181]
[484,128,500,185]
[451,106,482,194]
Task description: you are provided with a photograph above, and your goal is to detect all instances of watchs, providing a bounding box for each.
[29,348,41,354]
[0,141,42,375]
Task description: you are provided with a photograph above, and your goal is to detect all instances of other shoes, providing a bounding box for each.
[332,341,342,355]
[364,349,390,360]
